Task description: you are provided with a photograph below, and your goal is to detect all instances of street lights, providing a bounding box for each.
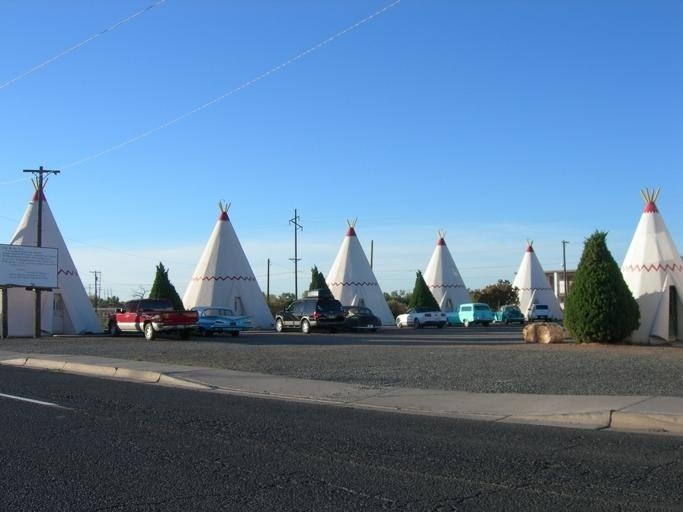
[561,239,569,297]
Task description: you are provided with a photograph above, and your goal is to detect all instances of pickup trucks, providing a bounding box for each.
[107,299,199,341]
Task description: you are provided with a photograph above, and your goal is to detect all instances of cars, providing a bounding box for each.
[395,305,447,329]
[189,305,255,337]
[493,304,525,326]
[340,306,381,333]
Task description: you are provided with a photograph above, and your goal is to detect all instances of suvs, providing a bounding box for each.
[275,299,346,334]
[445,303,493,329]
[527,304,552,322]
